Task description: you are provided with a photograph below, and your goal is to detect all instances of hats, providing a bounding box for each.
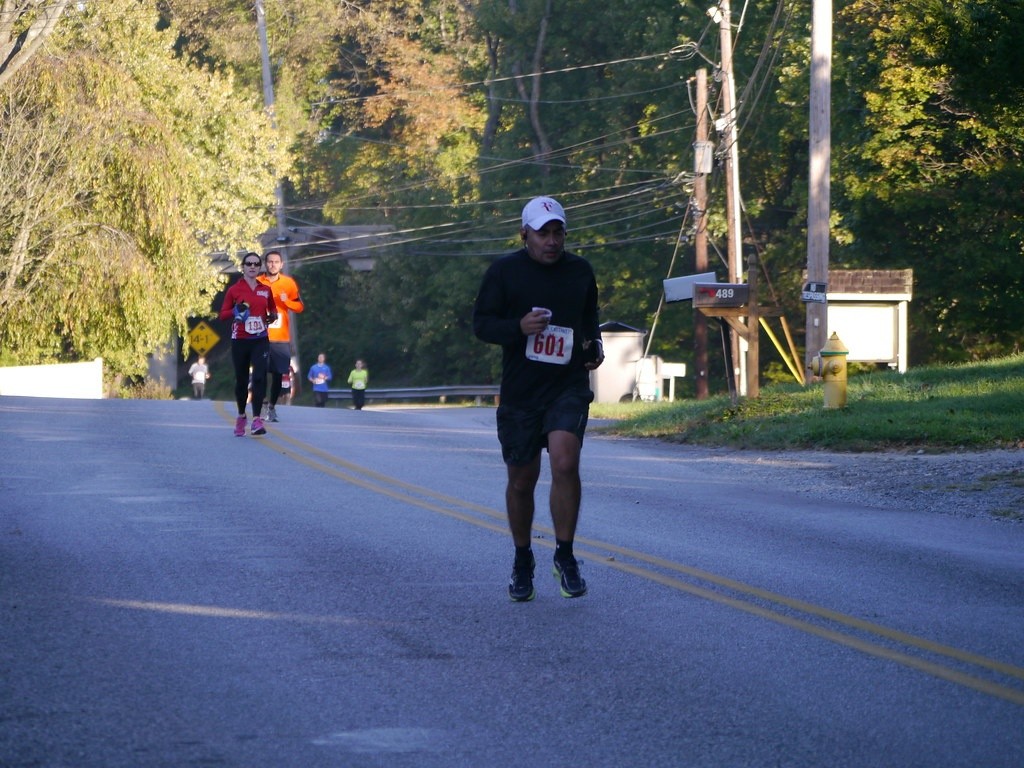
[522,196,566,231]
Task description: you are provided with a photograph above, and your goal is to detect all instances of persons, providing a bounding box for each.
[277,354,297,406]
[471,196,606,602]
[218,252,278,437]
[187,355,211,401]
[347,359,370,410]
[256,251,305,422]
[306,350,333,407]
[246,363,255,404]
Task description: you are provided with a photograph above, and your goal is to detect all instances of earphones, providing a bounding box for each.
[523,233,528,240]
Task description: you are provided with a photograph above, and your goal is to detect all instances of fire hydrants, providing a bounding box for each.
[807,330,850,410]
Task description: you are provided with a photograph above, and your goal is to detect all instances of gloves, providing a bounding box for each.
[237,300,247,312]
[266,314,275,324]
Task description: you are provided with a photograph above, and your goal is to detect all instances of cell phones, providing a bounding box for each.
[584,341,599,364]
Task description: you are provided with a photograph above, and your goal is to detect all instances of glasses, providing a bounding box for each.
[245,261,261,267]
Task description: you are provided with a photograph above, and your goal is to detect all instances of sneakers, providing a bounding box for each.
[260,404,266,420]
[251,418,266,435]
[266,406,279,422]
[552,554,587,598]
[234,412,247,436]
[509,552,536,601]
